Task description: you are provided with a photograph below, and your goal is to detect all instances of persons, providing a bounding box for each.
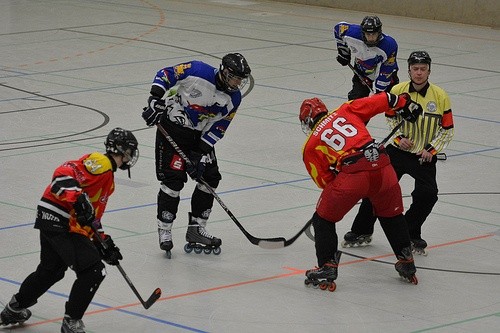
[0,127,141,333]
[343,52,453,249]
[140,54,252,250]
[299,92,417,278]
[334,16,400,101]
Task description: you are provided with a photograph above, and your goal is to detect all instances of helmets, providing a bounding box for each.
[299,97,328,127]
[219,52,252,92]
[407,50,432,65]
[106,127,140,170]
[360,15,382,34]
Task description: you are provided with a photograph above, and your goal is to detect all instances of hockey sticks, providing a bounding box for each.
[143,107,286,246]
[349,62,447,161]
[90,223,162,310]
[259,120,405,249]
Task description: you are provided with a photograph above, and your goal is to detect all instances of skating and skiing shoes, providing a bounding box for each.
[184,212,222,256]
[394,246,419,286]
[341,230,372,248]
[304,251,343,292]
[0,294,32,326]
[157,219,174,259]
[409,236,427,256]
[60,314,87,333]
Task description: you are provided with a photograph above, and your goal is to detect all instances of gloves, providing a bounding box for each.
[396,100,422,124]
[336,42,351,66]
[95,234,123,266]
[141,95,168,127]
[71,193,95,228]
[181,143,211,181]
